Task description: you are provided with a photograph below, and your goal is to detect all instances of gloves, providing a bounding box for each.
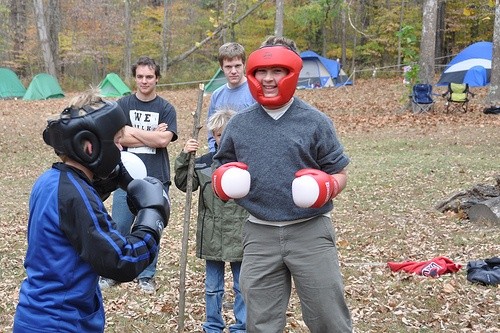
[126,175,171,240]
[97,151,147,193]
[291,169,339,209]
[212,162,250,202]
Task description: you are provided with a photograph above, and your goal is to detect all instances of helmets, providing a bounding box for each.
[246,46,304,109]
[42,98,126,182]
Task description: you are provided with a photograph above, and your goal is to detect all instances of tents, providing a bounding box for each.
[0,68,27,98]
[23,74,65,99]
[98,72,131,97]
[436,42,492,87]
[205,69,228,91]
[296,50,352,88]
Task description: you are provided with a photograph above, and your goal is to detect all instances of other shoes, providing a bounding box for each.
[99,277,115,288]
[137,279,157,296]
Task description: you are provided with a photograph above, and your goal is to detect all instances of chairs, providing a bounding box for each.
[407,83,441,116]
[442,82,477,114]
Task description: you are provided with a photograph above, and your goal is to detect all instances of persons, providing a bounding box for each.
[212,36,352,333]
[207,42,256,153]
[12,92,171,333]
[96,56,178,294]
[173,110,250,333]
